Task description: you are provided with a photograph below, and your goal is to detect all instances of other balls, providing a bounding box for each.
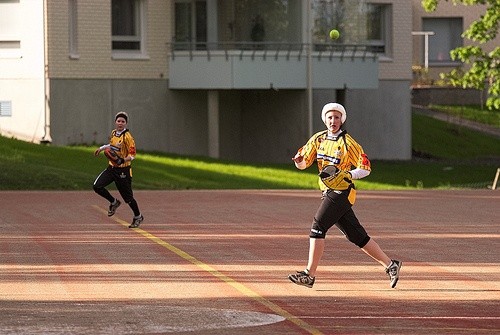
[329,29,340,40]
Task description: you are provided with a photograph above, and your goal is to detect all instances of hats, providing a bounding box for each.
[115,111,129,123]
[321,102,346,124]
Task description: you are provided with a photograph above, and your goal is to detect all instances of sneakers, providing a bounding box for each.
[108,198,121,217]
[384,259,402,288]
[288,269,315,288]
[129,214,144,228]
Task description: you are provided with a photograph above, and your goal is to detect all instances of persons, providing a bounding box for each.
[92,111,144,228]
[287,103,402,289]
